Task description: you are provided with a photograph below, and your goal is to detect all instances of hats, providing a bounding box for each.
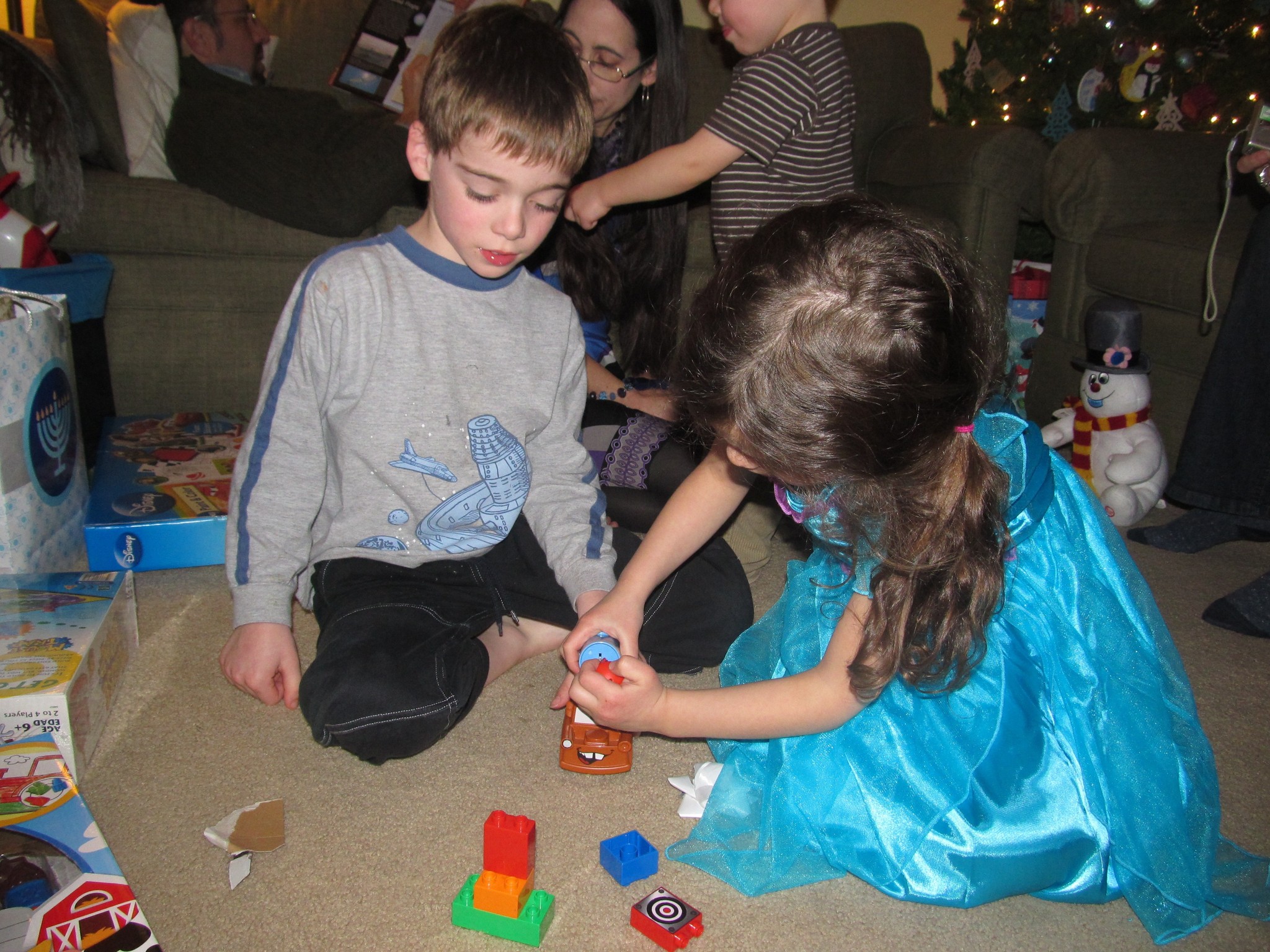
[1068,297,1154,375]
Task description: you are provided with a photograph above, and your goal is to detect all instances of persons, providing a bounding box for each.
[559,0,859,294]
[522,1,714,537]
[1126,150,1269,641]
[156,0,433,237]
[216,3,757,770]
[547,194,1268,947]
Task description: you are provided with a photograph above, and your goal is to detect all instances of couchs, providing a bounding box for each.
[4,3,474,421]
[1015,114,1256,493]
[674,17,1051,414]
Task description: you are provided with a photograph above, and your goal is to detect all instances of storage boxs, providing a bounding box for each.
[85,407,244,573]
[0,734,162,952]
[0,563,138,788]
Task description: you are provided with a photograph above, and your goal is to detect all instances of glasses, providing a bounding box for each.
[574,52,657,84]
[201,8,259,22]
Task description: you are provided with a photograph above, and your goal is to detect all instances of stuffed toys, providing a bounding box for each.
[1039,295,1170,527]
